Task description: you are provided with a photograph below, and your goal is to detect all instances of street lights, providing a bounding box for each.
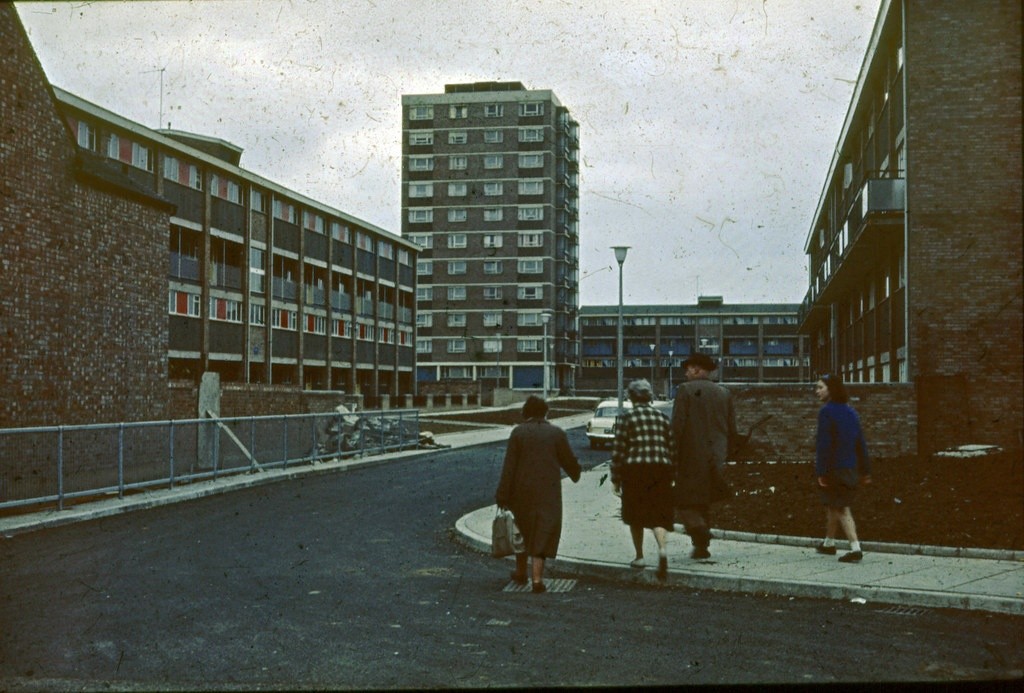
[609,246,632,415]
[668,350,674,399]
[649,344,656,405]
[701,339,708,355]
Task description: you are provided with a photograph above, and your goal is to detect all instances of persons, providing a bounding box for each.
[608,379,677,574]
[669,353,737,560]
[814,373,869,563]
[497,395,581,592]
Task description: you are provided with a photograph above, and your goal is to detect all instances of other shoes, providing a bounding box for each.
[692,531,712,560]
[509,570,527,587]
[658,549,667,573]
[532,581,544,592]
[815,541,836,554]
[631,557,645,567]
[839,549,862,563]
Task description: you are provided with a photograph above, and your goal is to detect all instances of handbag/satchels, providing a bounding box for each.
[491,503,526,558]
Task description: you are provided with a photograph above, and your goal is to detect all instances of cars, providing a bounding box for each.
[585,399,634,449]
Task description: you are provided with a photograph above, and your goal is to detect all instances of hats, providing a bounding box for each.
[680,352,715,371]
